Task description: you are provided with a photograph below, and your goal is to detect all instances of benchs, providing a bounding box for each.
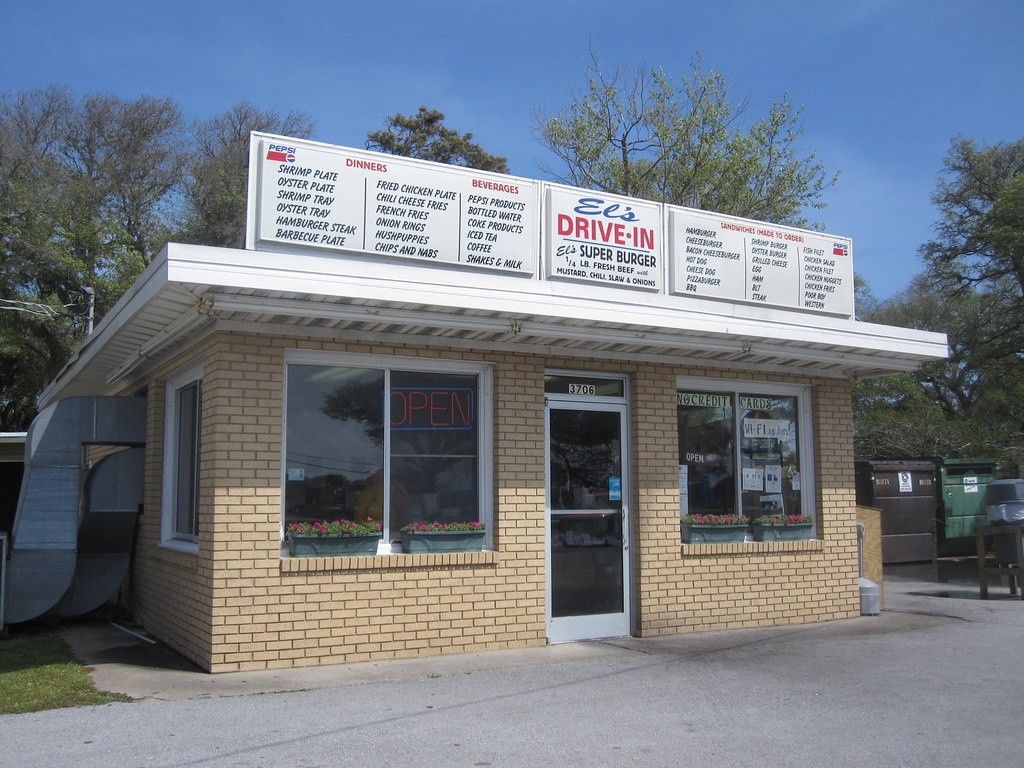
[933,554,1017,597]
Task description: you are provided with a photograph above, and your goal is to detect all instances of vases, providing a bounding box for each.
[401,530,487,554]
[752,523,813,542]
[681,524,750,543]
[286,531,382,557]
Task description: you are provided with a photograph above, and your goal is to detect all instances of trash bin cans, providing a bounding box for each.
[987,480,1024,565]
[855,458,937,562]
[932,455,1004,540]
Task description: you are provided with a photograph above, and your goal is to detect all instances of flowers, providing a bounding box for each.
[287,516,383,537]
[753,512,814,526]
[681,513,750,527]
[401,519,487,532]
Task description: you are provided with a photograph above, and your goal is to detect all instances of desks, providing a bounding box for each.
[974,524,1024,600]
